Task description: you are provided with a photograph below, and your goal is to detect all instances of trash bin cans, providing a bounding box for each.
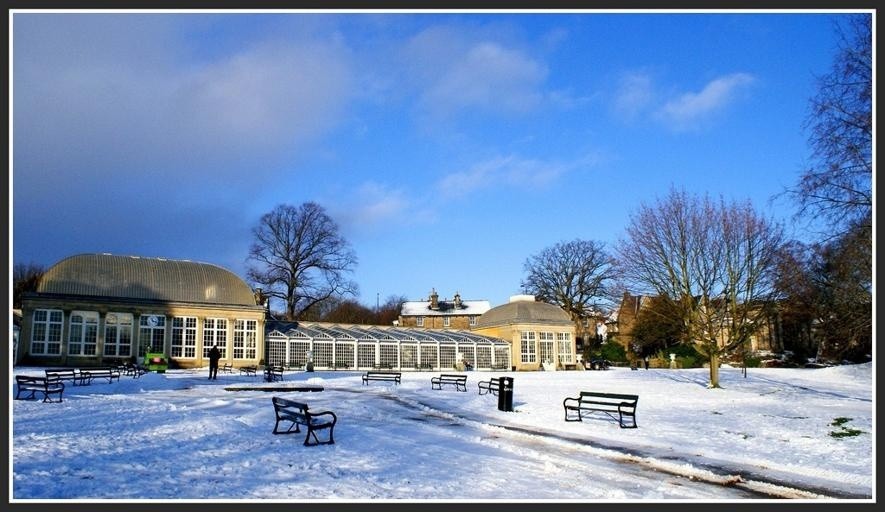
[498,377,513,411]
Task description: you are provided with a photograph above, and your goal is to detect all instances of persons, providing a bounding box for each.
[13,331,17,368]
[644,356,649,370]
[207,345,222,379]
[461,359,472,371]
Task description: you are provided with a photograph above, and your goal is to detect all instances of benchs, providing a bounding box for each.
[240,364,258,377]
[45,369,86,387]
[219,363,233,373]
[431,374,467,392]
[79,367,120,385]
[264,367,284,381]
[563,392,639,428]
[362,371,401,386]
[478,378,499,395]
[15,375,65,403]
[271,397,337,447]
